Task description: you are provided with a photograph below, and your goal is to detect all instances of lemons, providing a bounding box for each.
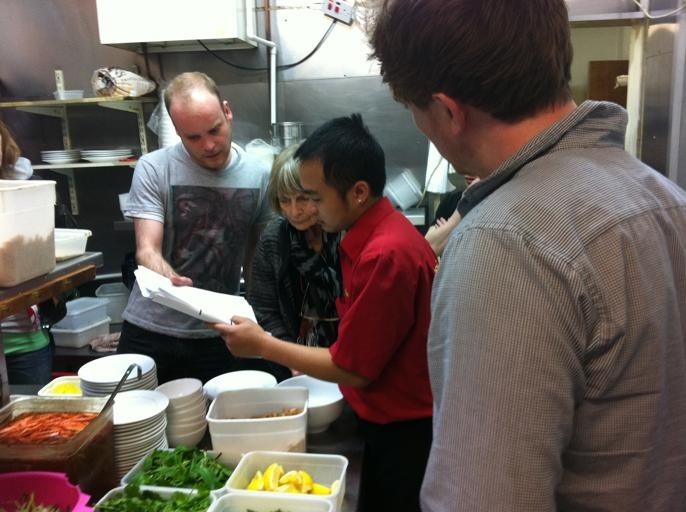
[244,464,341,493]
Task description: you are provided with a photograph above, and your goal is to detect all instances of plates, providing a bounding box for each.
[202,369,278,400]
[37,373,81,397]
[39,150,135,164]
[78,352,209,486]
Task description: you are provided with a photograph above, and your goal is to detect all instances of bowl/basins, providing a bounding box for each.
[0,471,95,512]
[279,374,344,434]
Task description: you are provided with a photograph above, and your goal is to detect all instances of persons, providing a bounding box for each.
[244,138,340,378]
[365,0,686,511]
[421,169,482,260]
[110,71,283,384]
[208,112,438,512]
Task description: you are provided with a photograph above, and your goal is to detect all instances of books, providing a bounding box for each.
[132,264,271,336]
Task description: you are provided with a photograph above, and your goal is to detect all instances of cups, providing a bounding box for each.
[270,122,302,150]
[387,172,423,211]
